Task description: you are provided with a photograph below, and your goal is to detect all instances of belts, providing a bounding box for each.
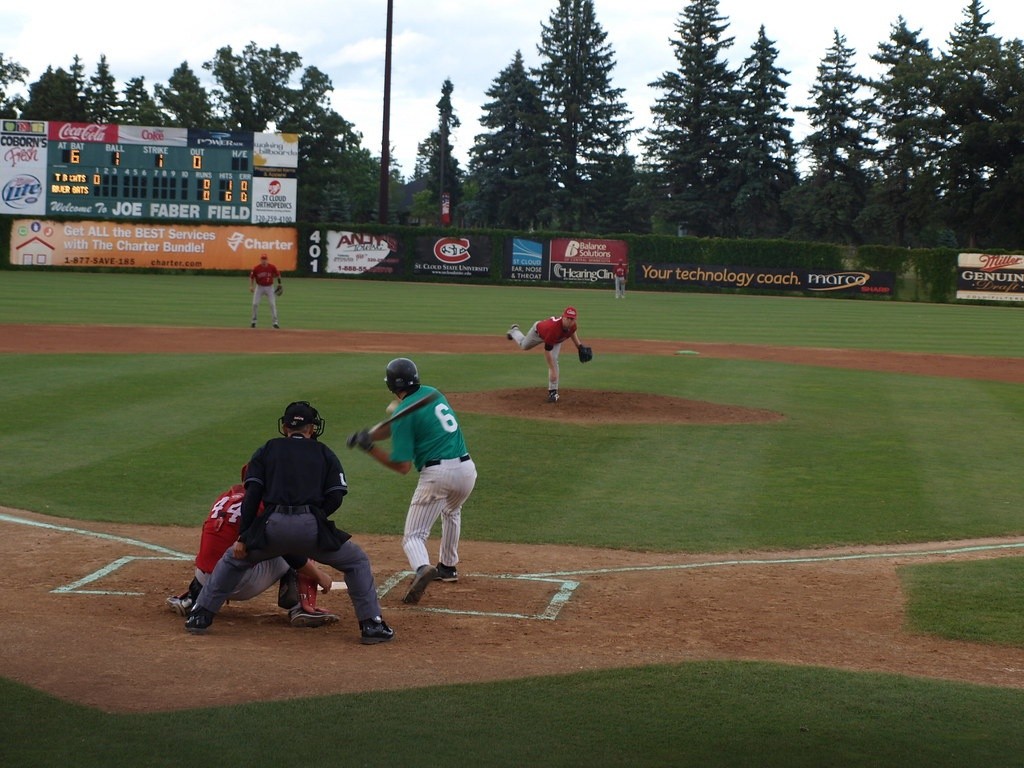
[425,455,470,467]
[272,504,311,514]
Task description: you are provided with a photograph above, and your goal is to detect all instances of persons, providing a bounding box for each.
[183,401,395,646]
[356,358,478,604]
[250,254,283,329]
[506,306,593,404]
[613,260,627,299]
[165,462,340,628]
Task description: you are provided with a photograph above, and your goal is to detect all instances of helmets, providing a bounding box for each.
[282,401,322,427]
[384,358,421,390]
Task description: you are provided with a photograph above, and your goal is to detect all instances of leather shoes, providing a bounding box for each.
[184,603,214,635]
[359,619,394,644]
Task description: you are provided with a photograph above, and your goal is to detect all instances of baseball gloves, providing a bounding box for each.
[578,346,593,362]
[275,286,283,295]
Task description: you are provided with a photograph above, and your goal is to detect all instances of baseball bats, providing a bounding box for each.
[366,392,439,436]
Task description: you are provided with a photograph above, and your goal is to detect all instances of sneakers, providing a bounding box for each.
[288,607,341,627]
[508,323,519,340]
[166,591,193,617]
[435,562,458,582]
[403,565,438,604]
[547,390,559,401]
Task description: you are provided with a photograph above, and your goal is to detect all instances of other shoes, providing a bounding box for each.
[251,323,255,328]
[273,325,279,329]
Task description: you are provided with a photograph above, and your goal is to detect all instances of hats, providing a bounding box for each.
[241,464,247,488]
[619,259,623,262]
[562,306,577,319]
[261,254,268,259]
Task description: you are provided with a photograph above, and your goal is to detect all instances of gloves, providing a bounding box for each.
[359,432,375,453]
